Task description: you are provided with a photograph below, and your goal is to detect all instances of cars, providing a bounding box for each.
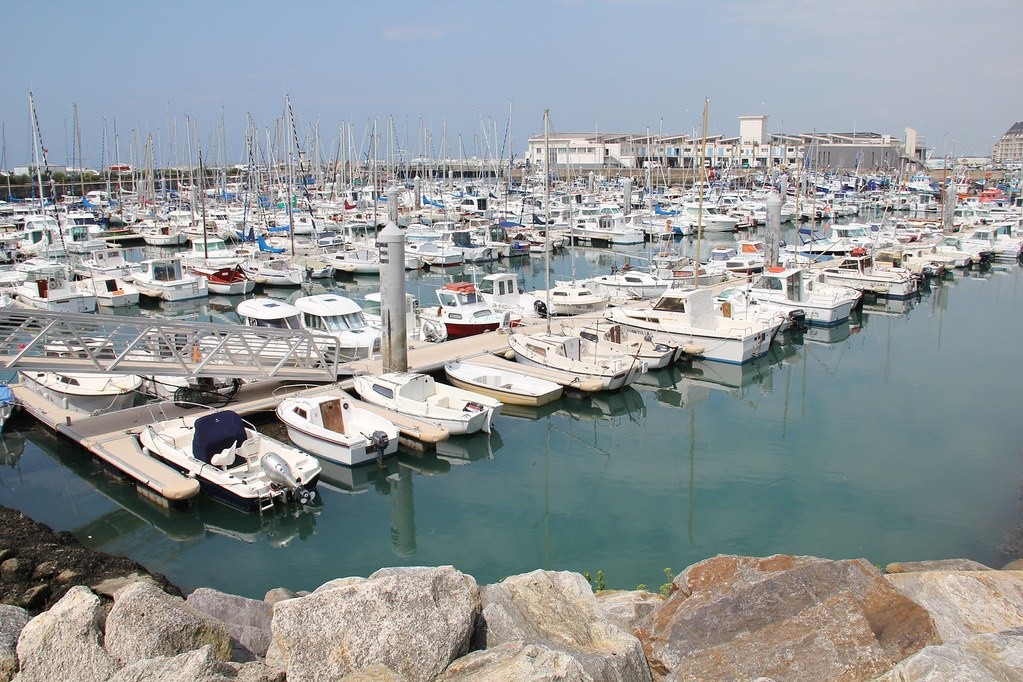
[742,163,749,168]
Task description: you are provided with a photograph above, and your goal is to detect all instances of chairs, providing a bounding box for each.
[237,435,265,472]
[211,442,240,474]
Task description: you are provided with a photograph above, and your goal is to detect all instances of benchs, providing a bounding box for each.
[501,382,513,389]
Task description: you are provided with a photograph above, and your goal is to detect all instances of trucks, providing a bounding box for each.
[644,161,661,170]
[704,160,710,168]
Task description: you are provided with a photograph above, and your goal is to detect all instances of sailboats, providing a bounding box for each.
[0,83,1023,518]
